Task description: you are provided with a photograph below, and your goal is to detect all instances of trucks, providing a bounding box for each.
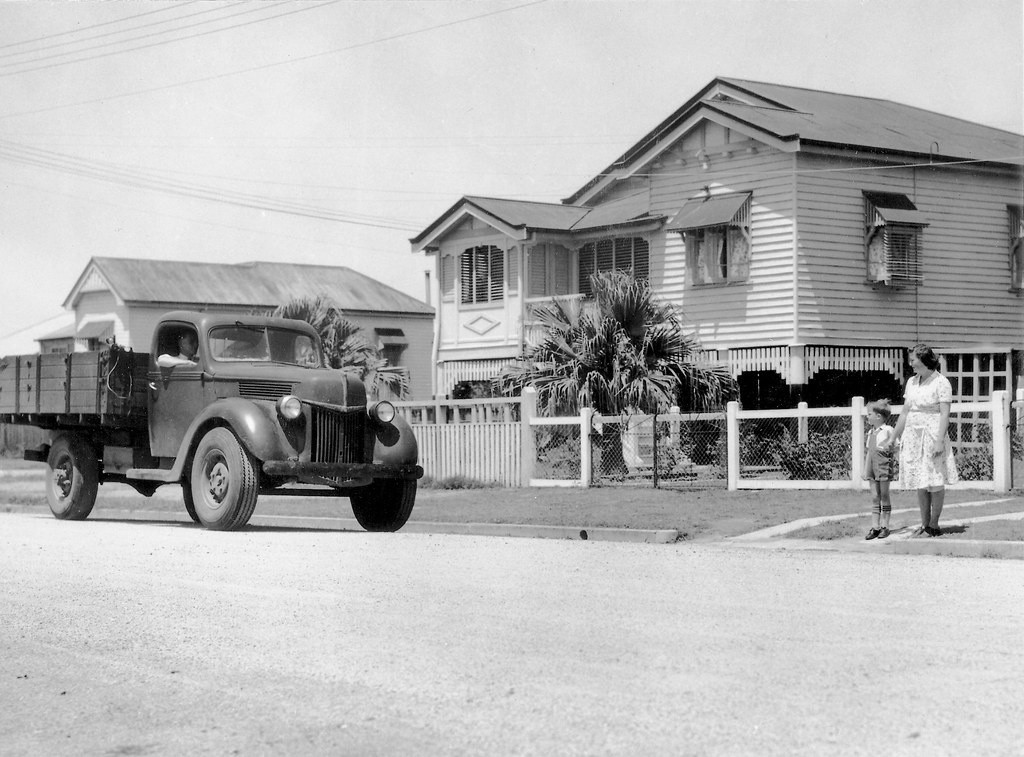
[0,310,424,532]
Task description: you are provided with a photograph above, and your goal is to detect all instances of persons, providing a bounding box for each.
[158,328,198,367]
[884,344,959,538]
[860,399,900,540]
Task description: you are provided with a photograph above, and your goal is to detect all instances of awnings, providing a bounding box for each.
[1006,203,1024,255]
[73,320,114,350]
[375,327,409,351]
[664,191,752,246]
[861,189,931,244]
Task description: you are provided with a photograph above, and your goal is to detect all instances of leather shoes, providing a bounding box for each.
[913,524,941,539]
[866,527,891,540]
